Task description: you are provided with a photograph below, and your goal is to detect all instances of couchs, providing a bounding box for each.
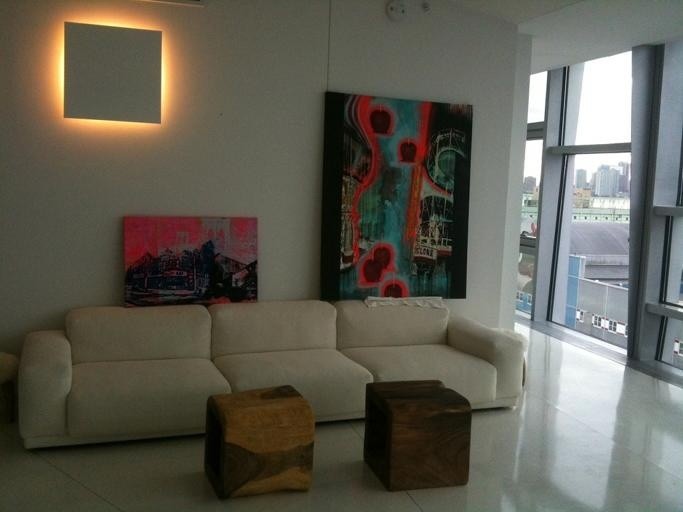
[18,297,523,449]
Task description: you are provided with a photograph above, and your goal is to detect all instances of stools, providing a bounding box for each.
[363,381,472,492]
[205,385,315,501]
